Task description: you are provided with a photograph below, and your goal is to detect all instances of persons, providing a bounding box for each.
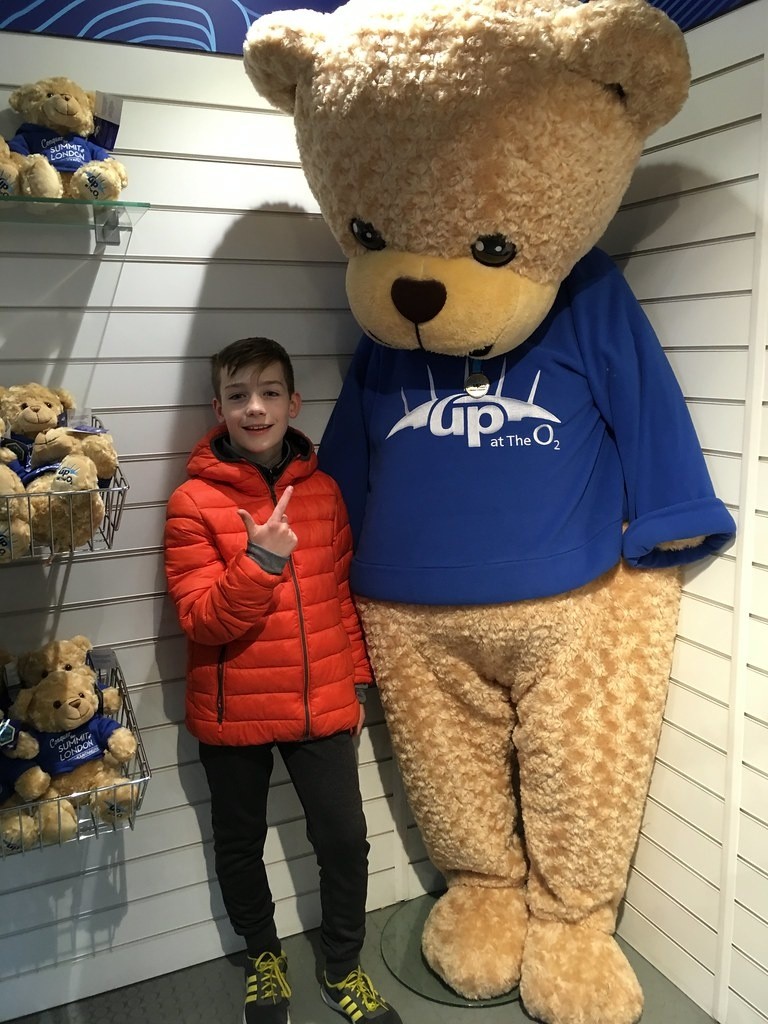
[167,338,404,1024]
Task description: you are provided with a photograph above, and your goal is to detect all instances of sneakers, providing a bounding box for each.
[242,949,292,1024]
[321,966,405,1024]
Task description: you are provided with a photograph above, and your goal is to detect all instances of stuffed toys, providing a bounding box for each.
[1,383,120,565]
[1,78,128,213]
[0,636,136,856]
[243,2,736,1024]
